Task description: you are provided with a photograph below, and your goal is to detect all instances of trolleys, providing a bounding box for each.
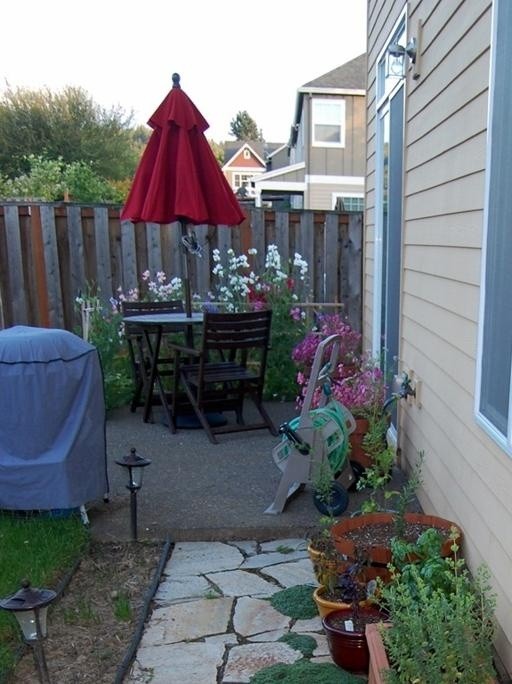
[260,332,371,518]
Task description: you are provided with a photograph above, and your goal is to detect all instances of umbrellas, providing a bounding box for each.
[119,72,249,317]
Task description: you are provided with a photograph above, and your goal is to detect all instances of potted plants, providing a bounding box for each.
[322,566,388,672]
[314,558,373,613]
[305,516,343,584]
[366,527,500,682]
[333,453,459,562]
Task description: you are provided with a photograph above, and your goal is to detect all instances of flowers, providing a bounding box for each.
[302,365,386,412]
[297,314,361,362]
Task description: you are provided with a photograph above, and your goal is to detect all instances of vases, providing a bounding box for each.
[349,415,387,465]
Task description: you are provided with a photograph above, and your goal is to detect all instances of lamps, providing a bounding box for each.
[385,35,417,80]
[0,578,57,684]
[114,446,152,542]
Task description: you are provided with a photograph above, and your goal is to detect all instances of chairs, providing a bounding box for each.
[120,299,279,444]
